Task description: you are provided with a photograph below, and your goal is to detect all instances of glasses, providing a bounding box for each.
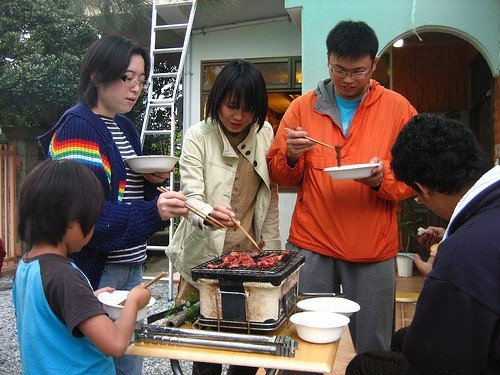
[120,75,151,90]
[326,53,374,80]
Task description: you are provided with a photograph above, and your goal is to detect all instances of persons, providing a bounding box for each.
[266,21,421,375]
[12,159,150,375]
[414,226,446,277]
[344,113,500,375]
[38,34,188,375]
[165,59,282,375]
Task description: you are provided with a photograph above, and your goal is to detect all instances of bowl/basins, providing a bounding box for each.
[296,296,361,317]
[324,163,380,180]
[289,311,350,344]
[99,290,155,322]
[125,155,179,173]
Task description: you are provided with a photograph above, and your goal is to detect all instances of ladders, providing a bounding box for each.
[140,0,198,302]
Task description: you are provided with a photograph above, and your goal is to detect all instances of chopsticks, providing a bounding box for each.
[118,271,166,306]
[156,185,227,231]
[230,215,261,251]
[284,128,333,150]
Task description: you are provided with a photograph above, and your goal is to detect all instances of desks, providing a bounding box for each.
[124,296,341,375]
[414,209,443,228]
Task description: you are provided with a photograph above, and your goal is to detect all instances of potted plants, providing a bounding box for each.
[396,201,422,278]
